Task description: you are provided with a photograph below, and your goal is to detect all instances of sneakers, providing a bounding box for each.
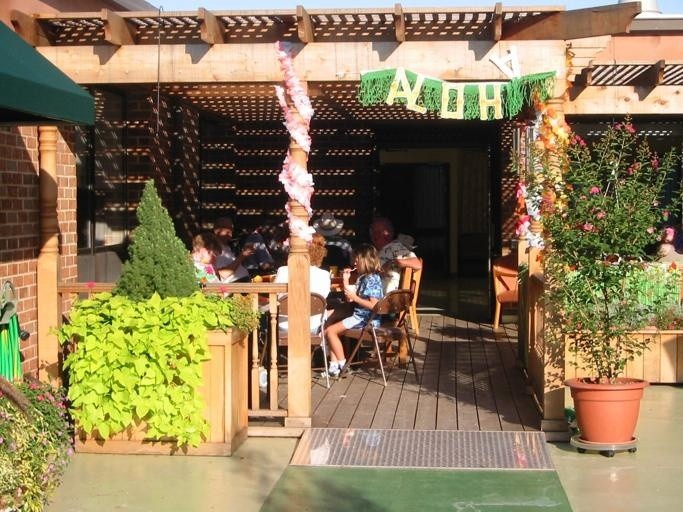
[320,365,351,379]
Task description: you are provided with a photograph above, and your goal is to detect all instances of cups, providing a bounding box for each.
[347,284,355,301]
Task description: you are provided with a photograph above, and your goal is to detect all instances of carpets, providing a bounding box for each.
[257,464,573,512]
[286,427,555,467]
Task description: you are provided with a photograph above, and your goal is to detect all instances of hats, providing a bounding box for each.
[313,213,344,236]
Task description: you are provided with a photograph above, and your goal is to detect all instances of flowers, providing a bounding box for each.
[509,115,682,383]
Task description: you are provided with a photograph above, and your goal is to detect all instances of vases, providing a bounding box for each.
[564,377,649,443]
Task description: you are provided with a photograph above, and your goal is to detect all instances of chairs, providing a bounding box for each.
[277,257,424,388]
[492,252,518,334]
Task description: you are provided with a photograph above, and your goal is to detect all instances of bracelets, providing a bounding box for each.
[389,259,395,270]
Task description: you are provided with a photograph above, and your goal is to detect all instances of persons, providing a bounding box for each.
[188,231,226,302]
[320,242,384,381]
[271,231,332,372]
[365,214,422,324]
[310,210,355,277]
[238,212,279,280]
[209,211,257,285]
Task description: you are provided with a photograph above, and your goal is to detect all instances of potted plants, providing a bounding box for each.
[45,179,261,456]
